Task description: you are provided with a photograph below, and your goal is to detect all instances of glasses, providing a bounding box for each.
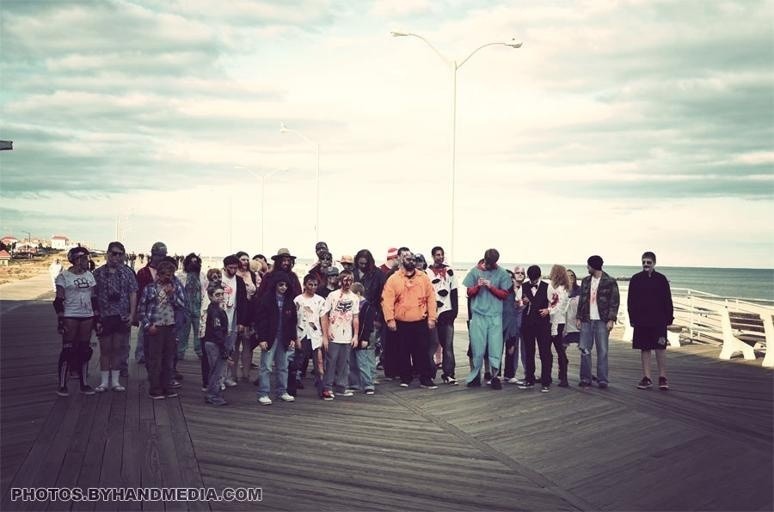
[514,272,525,276]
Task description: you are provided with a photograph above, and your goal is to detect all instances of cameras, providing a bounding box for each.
[108,292,120,302]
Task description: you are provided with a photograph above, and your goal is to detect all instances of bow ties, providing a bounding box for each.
[529,283,538,289]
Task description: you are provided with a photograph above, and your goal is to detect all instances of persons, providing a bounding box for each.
[627,252,674,390]
[54,242,617,406]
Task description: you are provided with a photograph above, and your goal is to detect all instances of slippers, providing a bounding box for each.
[111,384,126,392]
[95,385,108,392]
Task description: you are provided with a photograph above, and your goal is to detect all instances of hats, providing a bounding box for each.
[271,247,297,262]
[335,254,354,264]
[588,255,604,271]
[324,265,340,277]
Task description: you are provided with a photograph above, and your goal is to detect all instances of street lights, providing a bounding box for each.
[233,161,268,252]
[279,118,323,244]
[381,20,533,269]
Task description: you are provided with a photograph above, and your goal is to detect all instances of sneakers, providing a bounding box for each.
[658,376,670,390]
[55,390,69,396]
[149,370,184,400]
[201,377,236,403]
[256,372,608,406]
[637,375,654,389]
[80,385,96,395]
